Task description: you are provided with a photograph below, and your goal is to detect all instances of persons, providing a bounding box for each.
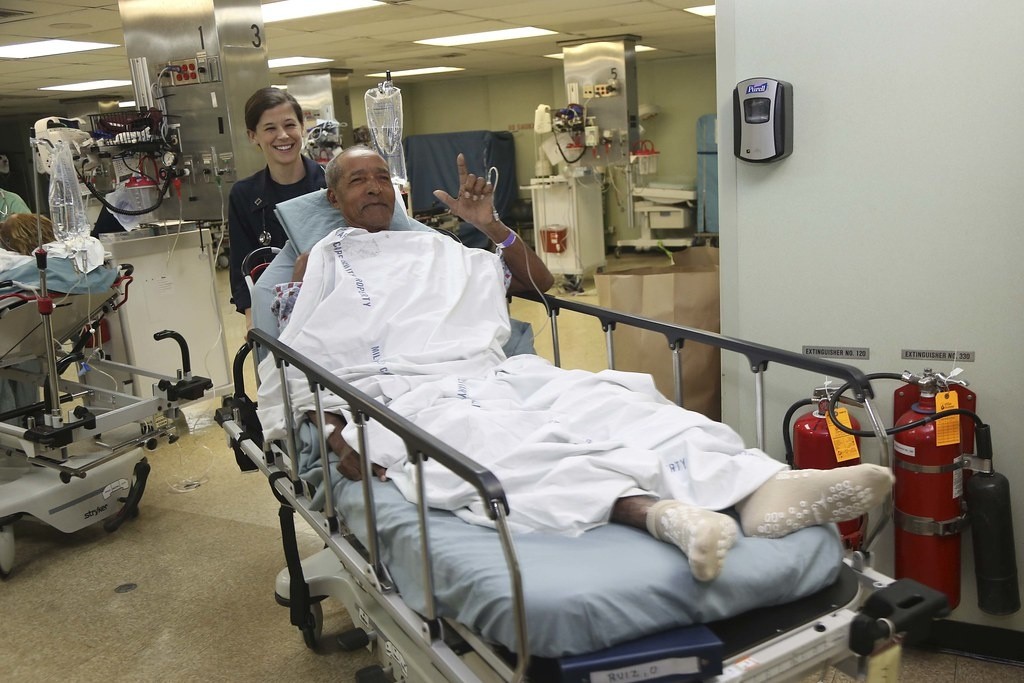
[271,145,895,581]
[0,187,58,430]
[228,87,331,353]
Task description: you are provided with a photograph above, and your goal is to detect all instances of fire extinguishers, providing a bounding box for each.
[784,349,1022,624]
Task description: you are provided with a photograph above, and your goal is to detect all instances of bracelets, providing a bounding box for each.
[495,230,516,249]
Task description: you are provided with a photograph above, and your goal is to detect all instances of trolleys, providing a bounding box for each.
[214,246,951,683]
[1,265,215,582]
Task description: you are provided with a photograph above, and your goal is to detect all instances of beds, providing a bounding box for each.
[0,248,234,577]
[217,246,947,682]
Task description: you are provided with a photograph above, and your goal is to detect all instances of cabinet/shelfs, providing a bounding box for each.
[71,227,236,433]
